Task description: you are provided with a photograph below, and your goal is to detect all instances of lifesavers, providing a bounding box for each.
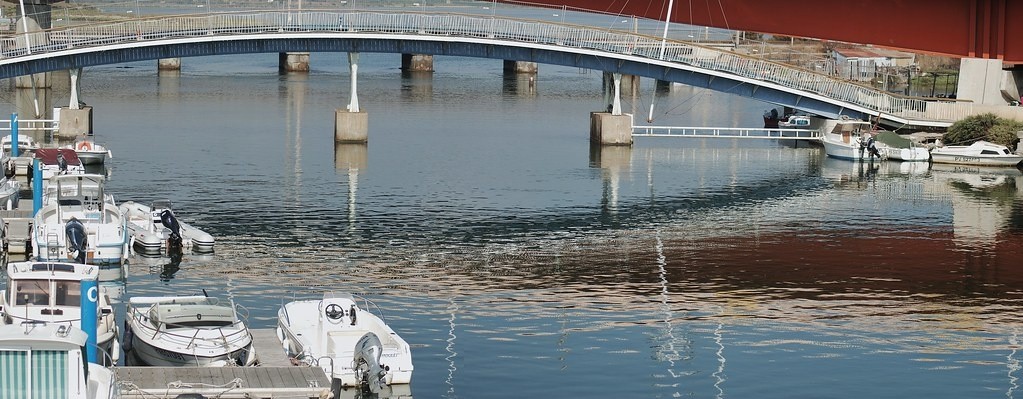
[79,142,91,152]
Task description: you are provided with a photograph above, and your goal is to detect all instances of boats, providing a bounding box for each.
[276,289,414,386]
[121,289,260,369]
[121,198,215,259]
[0,261,120,399]
[820,132,930,169]
[0,132,126,280]
[933,166,1023,193]
[929,139,1023,166]
[762,114,811,137]
